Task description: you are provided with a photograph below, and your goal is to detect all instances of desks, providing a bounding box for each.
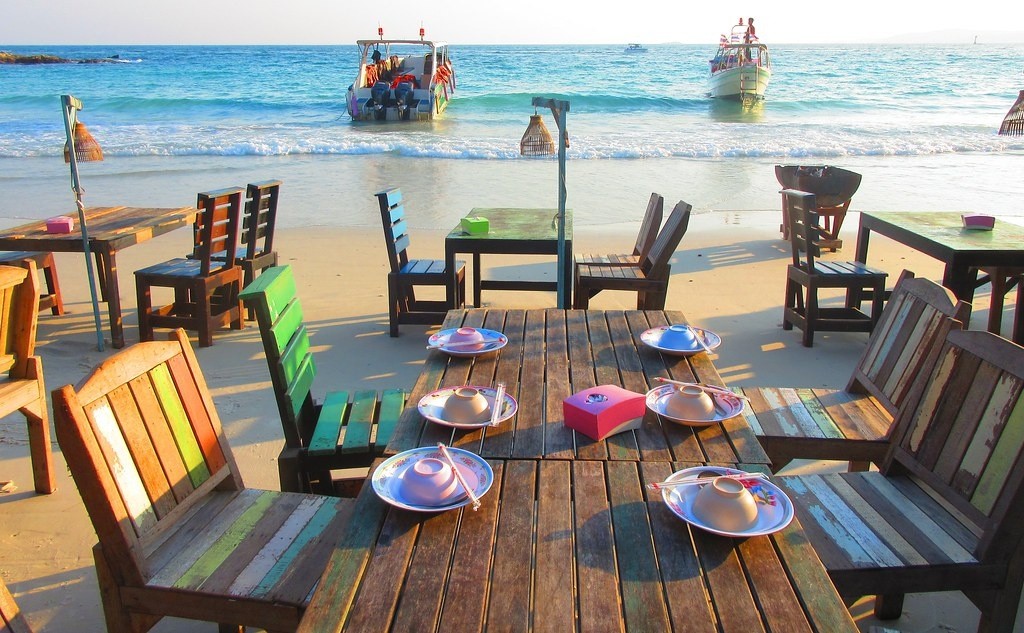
[445,206,575,309]
[0,207,205,347]
[853,211,1024,345]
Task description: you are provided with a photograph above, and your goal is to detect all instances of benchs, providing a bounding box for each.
[369,62,414,84]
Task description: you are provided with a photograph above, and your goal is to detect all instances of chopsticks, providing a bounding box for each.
[653,377,747,400]
[650,473,764,487]
[686,325,714,355]
[437,441,482,508]
[426,340,501,350]
[491,384,504,426]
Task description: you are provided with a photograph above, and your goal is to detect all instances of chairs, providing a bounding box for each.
[576,200,692,310]
[133,185,246,348]
[374,186,466,338]
[779,188,890,347]
[186,179,282,320]
[238,264,411,497]
[0,258,56,494]
[773,317,1024,633]
[573,193,665,310]
[0,251,63,316]
[51,328,356,633]
[726,269,973,475]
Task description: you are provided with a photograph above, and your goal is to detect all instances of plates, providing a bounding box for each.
[427,329,508,357]
[662,466,794,536]
[640,327,721,355]
[372,446,493,511]
[645,383,745,426]
[417,386,518,430]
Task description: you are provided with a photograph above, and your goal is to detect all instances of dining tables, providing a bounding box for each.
[297,456,859,632]
[381,309,773,464]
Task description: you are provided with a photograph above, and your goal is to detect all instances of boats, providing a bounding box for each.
[622,42,648,54]
[345,25,457,121]
[707,18,771,96]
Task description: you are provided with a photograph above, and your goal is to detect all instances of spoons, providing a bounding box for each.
[404,490,474,508]
[698,470,763,503]
[698,382,727,416]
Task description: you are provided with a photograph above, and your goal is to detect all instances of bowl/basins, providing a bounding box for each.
[658,325,698,350]
[666,385,716,421]
[693,476,759,532]
[401,326,491,506]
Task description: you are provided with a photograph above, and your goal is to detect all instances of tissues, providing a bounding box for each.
[459,214,490,235]
[562,384,647,443]
